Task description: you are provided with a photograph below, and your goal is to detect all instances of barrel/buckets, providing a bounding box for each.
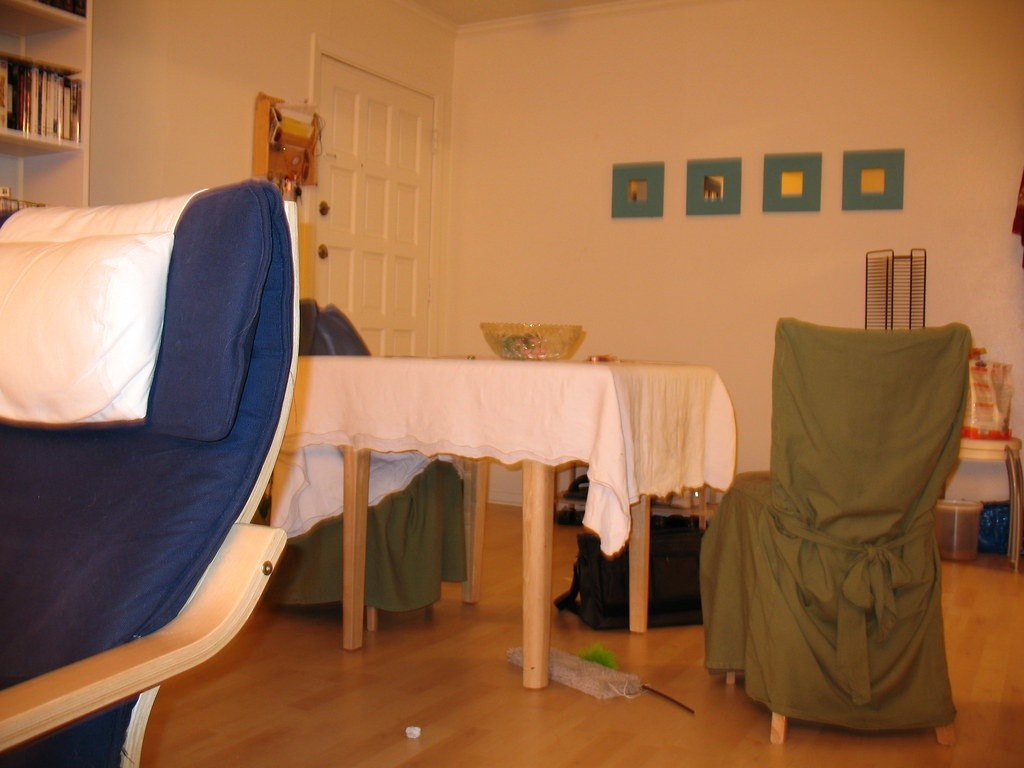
[936,500,983,562]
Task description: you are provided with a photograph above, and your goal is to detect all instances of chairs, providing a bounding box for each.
[697,317,974,745]
[271,297,466,634]
[0,179,302,768]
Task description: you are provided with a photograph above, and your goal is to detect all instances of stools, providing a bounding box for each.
[942,437,1024,568]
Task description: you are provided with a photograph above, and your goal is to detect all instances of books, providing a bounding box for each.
[0,196,46,228]
[0,55,83,143]
[38,0,87,17]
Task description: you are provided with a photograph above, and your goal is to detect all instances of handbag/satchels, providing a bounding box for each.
[553,512,704,631]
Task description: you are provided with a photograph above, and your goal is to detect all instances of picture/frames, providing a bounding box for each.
[687,157,742,215]
[611,162,665,217]
[841,149,904,210]
[763,151,822,213]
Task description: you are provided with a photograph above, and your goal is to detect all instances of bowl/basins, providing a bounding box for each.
[479,322,582,361]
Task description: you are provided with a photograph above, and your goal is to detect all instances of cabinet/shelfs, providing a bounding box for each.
[0,0,96,228]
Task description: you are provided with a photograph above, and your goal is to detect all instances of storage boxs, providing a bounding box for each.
[935,500,983,560]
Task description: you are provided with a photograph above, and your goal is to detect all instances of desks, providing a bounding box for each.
[267,355,739,689]
